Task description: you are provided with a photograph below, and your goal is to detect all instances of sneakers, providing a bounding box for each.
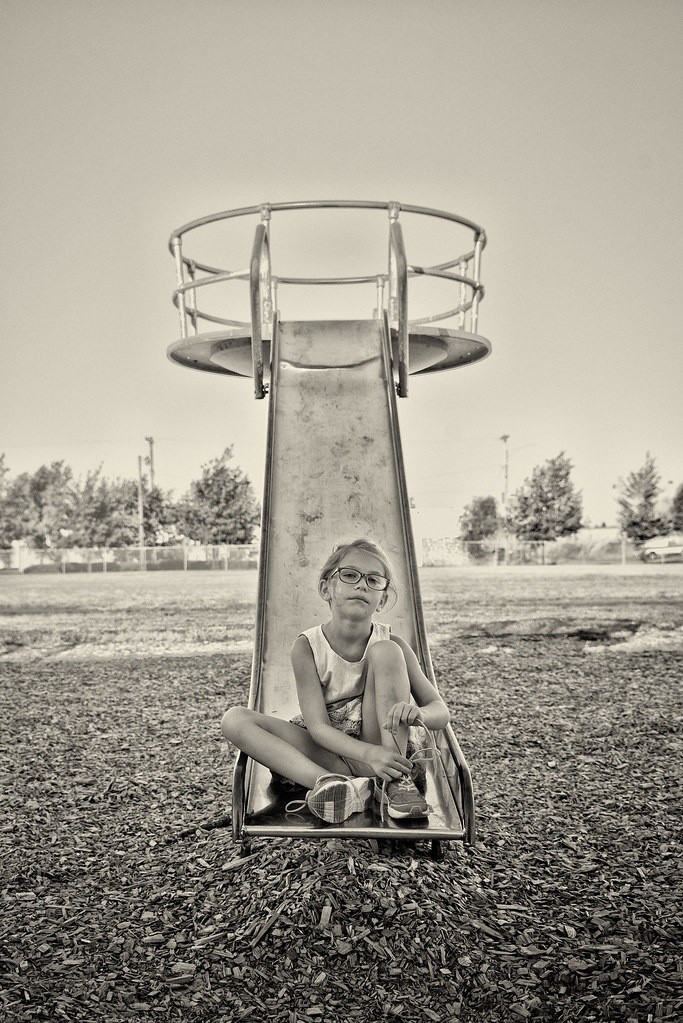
[306,774,376,823]
[372,771,433,820]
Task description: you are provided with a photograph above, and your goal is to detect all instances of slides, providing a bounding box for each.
[162,195,493,864]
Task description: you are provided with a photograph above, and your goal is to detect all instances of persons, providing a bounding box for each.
[219,537,450,824]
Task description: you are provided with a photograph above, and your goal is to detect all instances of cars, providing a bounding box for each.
[641,534,682,564]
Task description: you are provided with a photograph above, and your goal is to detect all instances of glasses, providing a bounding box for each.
[325,565,392,591]
